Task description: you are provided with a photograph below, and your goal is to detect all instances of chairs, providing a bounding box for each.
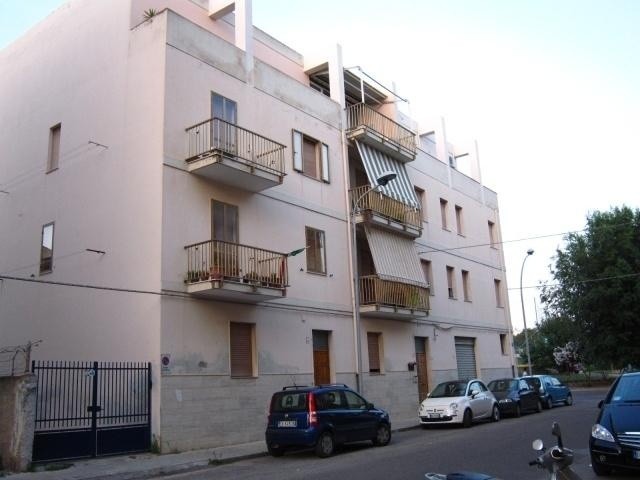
[325,394,340,408]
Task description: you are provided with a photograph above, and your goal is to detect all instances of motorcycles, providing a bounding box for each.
[422,416,581,479]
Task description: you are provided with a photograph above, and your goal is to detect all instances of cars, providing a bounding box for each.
[265,382,393,457]
[416,372,577,426]
[587,370,640,480]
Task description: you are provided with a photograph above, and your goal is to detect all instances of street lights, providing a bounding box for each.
[520,248,536,375]
[349,170,398,398]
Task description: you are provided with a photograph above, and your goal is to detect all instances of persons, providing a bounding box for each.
[522,368,528,376]
